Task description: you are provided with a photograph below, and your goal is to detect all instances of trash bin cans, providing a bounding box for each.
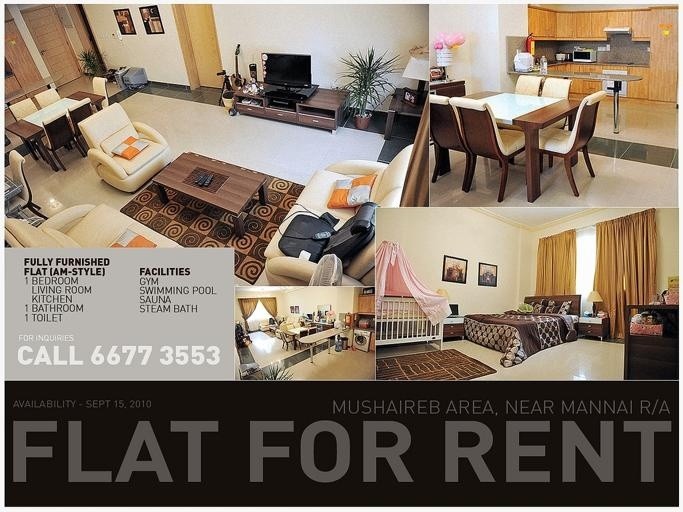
[341,337,350,350]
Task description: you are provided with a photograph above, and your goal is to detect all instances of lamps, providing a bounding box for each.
[436,47,453,82]
[586,291,603,316]
[401,56,430,104]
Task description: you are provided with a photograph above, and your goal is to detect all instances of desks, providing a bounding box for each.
[385,84,429,140]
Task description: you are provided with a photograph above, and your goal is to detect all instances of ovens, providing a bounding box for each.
[601,69,627,97]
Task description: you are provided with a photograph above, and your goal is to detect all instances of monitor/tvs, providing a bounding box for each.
[123,67,148,87]
[450,304,459,315]
[261,52,311,91]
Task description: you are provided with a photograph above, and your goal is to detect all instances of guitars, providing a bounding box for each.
[231,44,246,91]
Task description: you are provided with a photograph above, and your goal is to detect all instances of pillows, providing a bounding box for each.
[328,173,378,209]
[531,299,571,315]
[111,135,149,160]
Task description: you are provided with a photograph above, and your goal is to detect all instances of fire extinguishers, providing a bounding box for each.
[527,32,536,57]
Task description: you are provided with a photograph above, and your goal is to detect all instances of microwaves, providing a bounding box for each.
[572,50,596,63]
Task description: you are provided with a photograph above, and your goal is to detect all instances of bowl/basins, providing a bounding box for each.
[555,54,565,61]
[564,53,572,61]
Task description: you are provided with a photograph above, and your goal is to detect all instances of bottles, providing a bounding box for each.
[539,56,547,75]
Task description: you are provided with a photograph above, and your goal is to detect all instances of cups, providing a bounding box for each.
[241,82,264,96]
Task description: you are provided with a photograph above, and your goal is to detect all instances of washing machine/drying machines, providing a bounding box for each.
[352,330,372,353]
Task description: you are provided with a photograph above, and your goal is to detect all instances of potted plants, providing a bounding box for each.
[332,45,405,129]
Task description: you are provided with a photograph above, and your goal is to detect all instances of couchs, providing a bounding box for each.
[264,145,414,286]
[5,205,181,247]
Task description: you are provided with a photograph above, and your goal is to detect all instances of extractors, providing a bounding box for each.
[603,27,631,35]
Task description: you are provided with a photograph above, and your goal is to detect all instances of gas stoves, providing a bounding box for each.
[603,61,633,65]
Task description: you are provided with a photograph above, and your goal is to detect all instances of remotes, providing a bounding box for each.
[195,173,205,182]
[205,174,213,186]
[198,175,207,186]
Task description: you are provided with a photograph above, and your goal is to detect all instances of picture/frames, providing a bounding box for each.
[478,262,498,287]
[441,254,468,284]
[139,5,164,35]
[113,8,136,35]
[290,306,299,313]
[430,68,443,82]
[402,86,417,108]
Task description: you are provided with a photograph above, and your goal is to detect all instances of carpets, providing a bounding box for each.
[376,349,498,380]
[121,151,305,286]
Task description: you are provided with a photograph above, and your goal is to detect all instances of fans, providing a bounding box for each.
[306,253,343,286]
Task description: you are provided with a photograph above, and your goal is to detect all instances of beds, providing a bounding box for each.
[151,151,268,237]
[464,295,580,367]
[375,240,453,352]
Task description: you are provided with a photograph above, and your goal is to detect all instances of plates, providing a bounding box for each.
[532,69,539,72]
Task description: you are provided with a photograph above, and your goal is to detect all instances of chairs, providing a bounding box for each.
[282,323,316,351]
[5,76,109,219]
[77,101,174,193]
[429,73,606,203]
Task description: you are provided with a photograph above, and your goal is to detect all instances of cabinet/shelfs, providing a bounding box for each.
[359,295,375,314]
[235,80,351,134]
[323,324,337,339]
[528,5,679,105]
[625,305,679,380]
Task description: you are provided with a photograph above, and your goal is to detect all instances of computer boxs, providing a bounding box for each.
[114,67,129,88]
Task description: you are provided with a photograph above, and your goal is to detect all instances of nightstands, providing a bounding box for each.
[443,318,463,341]
[579,316,610,341]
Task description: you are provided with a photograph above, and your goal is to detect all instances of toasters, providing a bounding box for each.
[512,52,534,73]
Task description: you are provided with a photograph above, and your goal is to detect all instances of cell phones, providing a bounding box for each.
[310,231,330,240]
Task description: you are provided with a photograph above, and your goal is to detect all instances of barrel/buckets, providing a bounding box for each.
[539,55,549,74]
